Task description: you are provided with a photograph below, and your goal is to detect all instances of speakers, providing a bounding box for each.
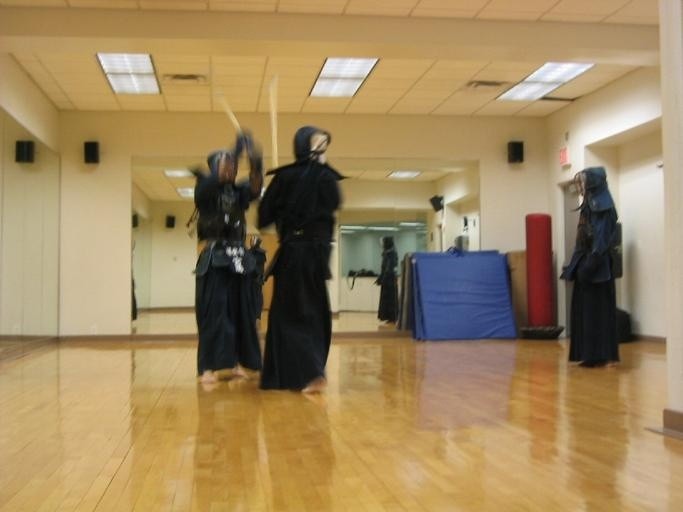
[84,141,98,162]
[133,214,137,227]
[508,141,523,162]
[15,140,33,162]
[430,195,441,211]
[166,215,176,228]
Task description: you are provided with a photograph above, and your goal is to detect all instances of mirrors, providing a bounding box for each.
[130,151,480,335]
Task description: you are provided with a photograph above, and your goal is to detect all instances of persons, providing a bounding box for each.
[374,236,399,324]
[257,126,351,392]
[194,127,262,384]
[559,167,620,368]
[248,235,266,320]
